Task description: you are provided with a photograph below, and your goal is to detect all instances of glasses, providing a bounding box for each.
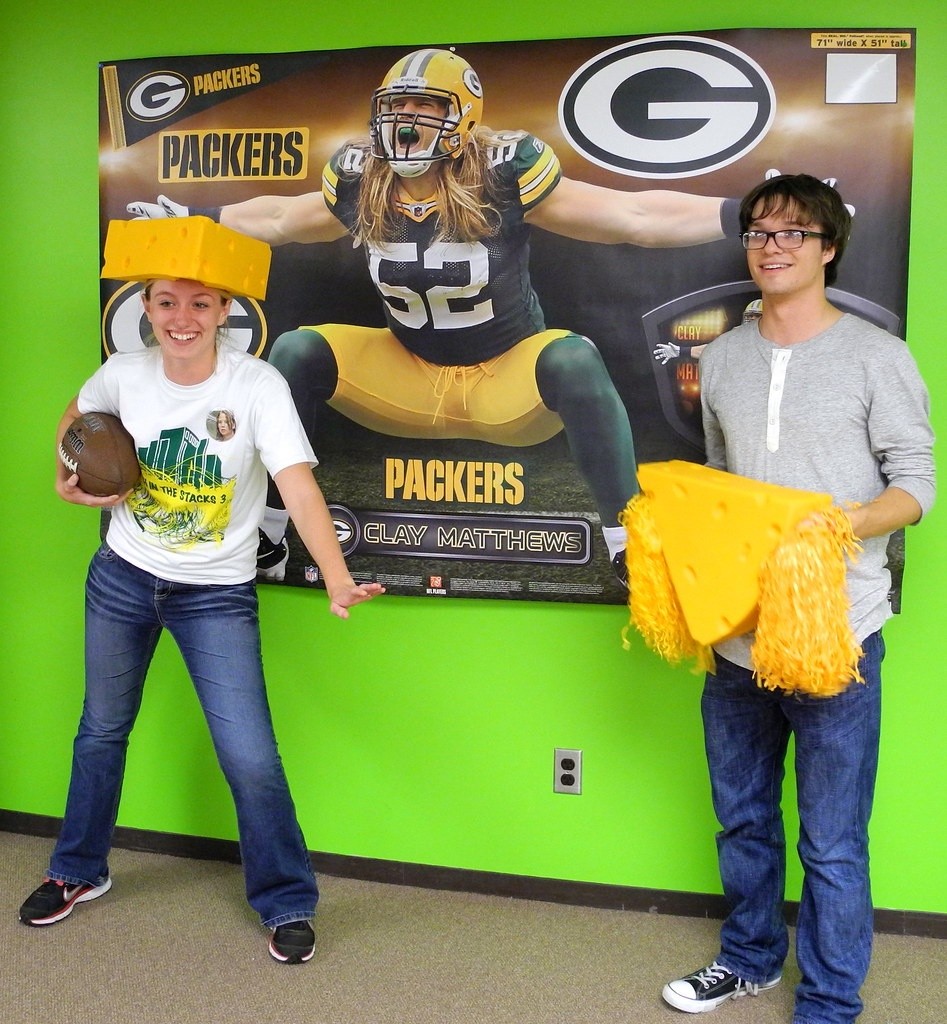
[739,229,826,250]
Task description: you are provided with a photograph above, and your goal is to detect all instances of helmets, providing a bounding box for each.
[371,48,484,160]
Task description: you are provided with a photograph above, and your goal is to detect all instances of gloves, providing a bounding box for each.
[764,169,856,220]
[127,194,189,220]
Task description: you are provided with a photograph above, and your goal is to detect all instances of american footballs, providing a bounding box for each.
[58,412,140,497]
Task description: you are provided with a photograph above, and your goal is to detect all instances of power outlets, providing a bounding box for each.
[553,749,583,795]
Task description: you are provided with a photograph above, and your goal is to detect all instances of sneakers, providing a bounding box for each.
[662,960,781,1013]
[19,872,113,927]
[611,548,629,587]
[268,920,316,963]
[256,527,289,581]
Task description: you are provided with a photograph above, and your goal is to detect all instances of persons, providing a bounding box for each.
[120,49,860,591]
[653,297,763,366]
[624,172,937,1023]
[15,214,392,962]
